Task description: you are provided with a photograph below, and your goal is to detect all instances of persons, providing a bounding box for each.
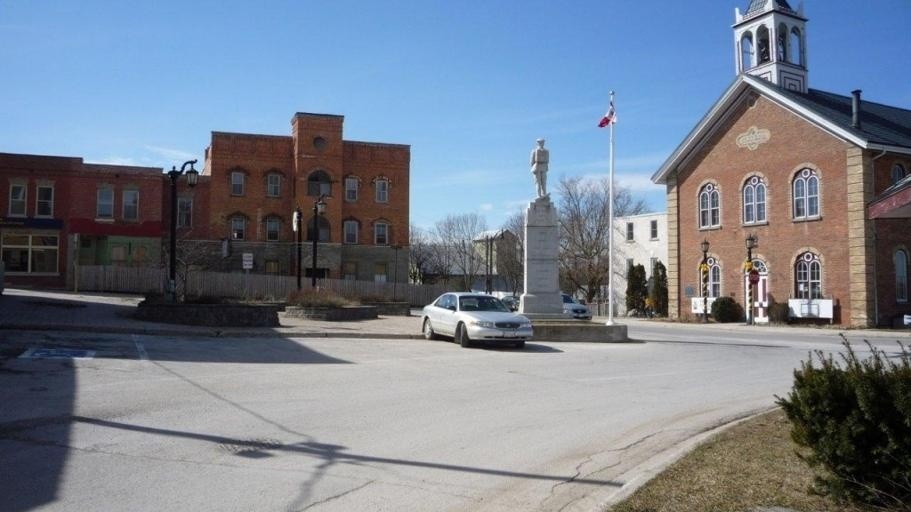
[530,138,549,198]
[636,291,654,319]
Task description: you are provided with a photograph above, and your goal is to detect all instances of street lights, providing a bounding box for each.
[745,233,754,325]
[700,236,710,323]
[166,158,199,301]
[295,193,327,288]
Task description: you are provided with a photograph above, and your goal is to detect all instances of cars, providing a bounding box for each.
[561,293,592,320]
[420,291,533,348]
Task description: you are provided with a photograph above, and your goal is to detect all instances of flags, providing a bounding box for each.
[597,101,616,128]
